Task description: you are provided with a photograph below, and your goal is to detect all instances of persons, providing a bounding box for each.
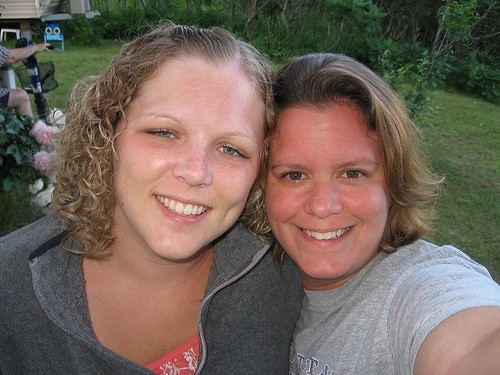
[237,53,500,375]
[0,26,304,375]
[0,40,50,124]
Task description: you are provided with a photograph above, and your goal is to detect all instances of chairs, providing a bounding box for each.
[42,22,65,52]
